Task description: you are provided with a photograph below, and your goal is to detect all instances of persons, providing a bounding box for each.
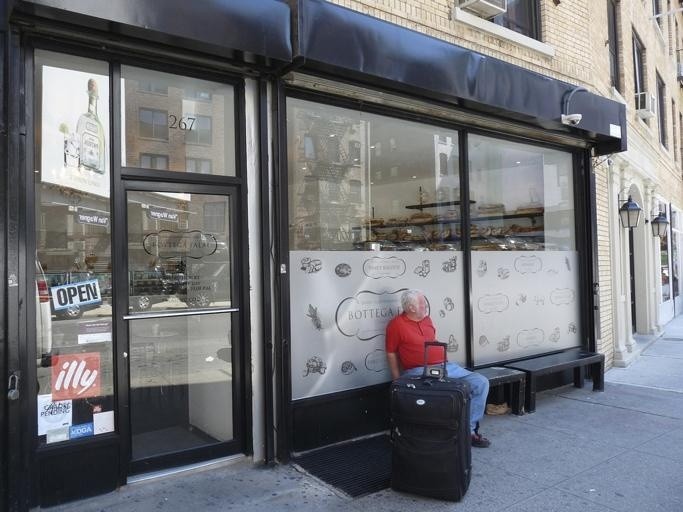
[385,289,490,449]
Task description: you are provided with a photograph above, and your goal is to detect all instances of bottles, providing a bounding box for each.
[61,78,108,176]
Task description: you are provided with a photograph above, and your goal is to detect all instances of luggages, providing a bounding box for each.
[386,339,473,503]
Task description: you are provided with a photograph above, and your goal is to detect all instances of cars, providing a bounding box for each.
[306,232,550,252]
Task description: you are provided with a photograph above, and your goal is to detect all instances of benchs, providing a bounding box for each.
[474,347,605,413]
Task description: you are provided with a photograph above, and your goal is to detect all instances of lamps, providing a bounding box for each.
[649,211,668,237]
[616,193,640,228]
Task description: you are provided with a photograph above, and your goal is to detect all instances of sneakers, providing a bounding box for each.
[471,432,490,447]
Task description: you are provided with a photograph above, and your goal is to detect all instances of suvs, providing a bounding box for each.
[35,259,57,395]
[52,241,231,317]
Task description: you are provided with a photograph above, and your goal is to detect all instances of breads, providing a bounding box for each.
[358,202,543,251]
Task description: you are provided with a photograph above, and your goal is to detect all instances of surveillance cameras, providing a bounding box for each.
[560,114,582,125]
